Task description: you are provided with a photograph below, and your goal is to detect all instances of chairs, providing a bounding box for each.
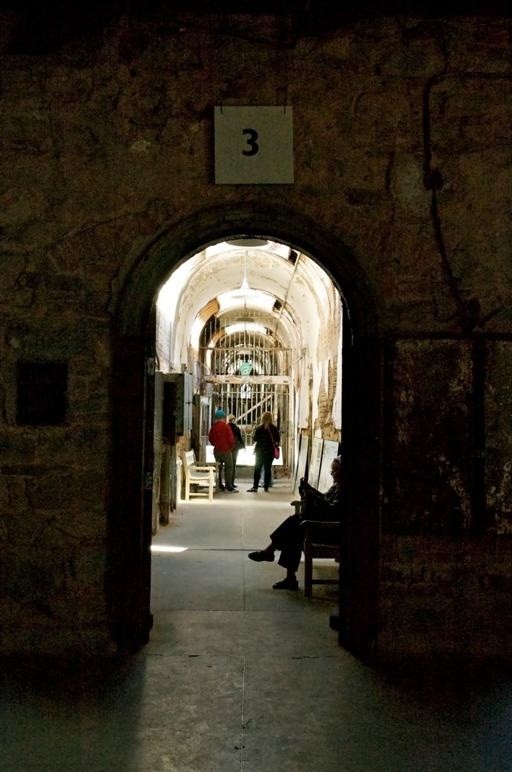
[289,496,344,596]
[181,449,219,496]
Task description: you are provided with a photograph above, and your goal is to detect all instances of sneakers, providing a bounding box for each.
[273,577,298,590]
[249,551,274,562]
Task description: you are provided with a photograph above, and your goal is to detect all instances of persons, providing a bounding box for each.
[224,414,245,488]
[247,413,282,492]
[208,410,238,493]
[249,454,342,590]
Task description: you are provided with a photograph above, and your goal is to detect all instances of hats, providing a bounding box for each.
[215,410,225,417]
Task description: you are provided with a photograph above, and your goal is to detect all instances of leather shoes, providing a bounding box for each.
[219,483,272,492]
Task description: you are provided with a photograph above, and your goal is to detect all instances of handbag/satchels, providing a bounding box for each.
[274,447,280,459]
